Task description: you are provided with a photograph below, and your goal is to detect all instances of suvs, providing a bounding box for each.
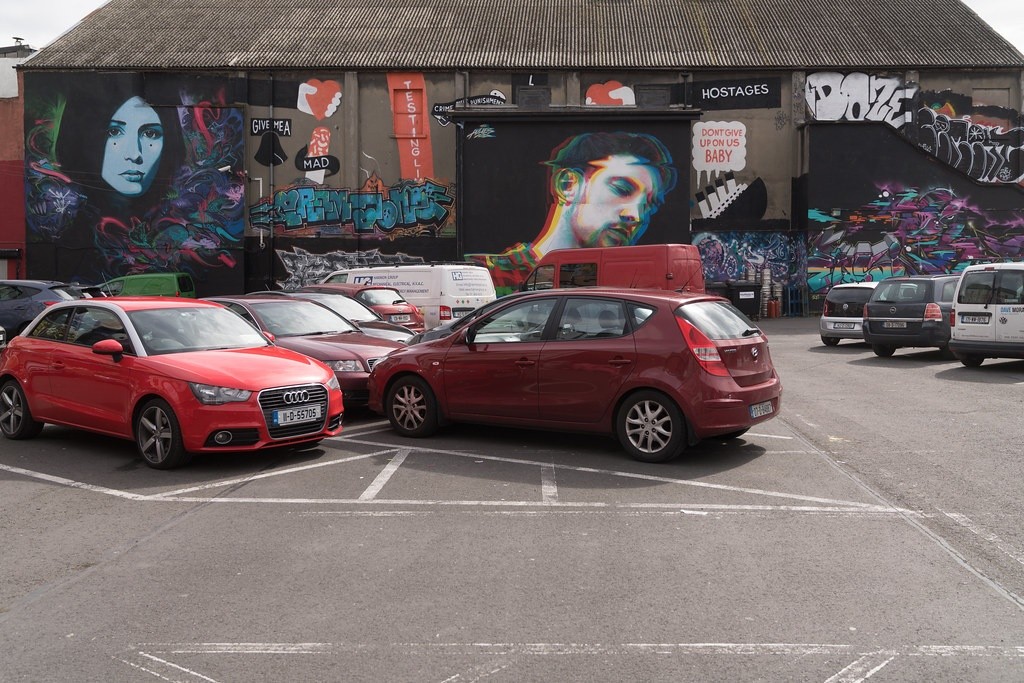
[0,279,109,352]
[861,274,959,362]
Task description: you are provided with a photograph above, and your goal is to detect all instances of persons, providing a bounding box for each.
[463,128,678,300]
[47,71,186,228]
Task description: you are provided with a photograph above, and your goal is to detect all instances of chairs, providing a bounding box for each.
[596,310,623,337]
[558,307,587,339]
[901,288,916,301]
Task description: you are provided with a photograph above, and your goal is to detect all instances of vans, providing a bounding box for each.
[96,274,197,301]
[319,265,497,330]
[512,244,708,294]
[949,261,1024,369]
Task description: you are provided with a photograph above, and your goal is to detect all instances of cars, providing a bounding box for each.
[290,283,426,334]
[0,294,345,470]
[366,286,783,464]
[250,290,417,345]
[819,281,880,347]
[195,294,409,421]
